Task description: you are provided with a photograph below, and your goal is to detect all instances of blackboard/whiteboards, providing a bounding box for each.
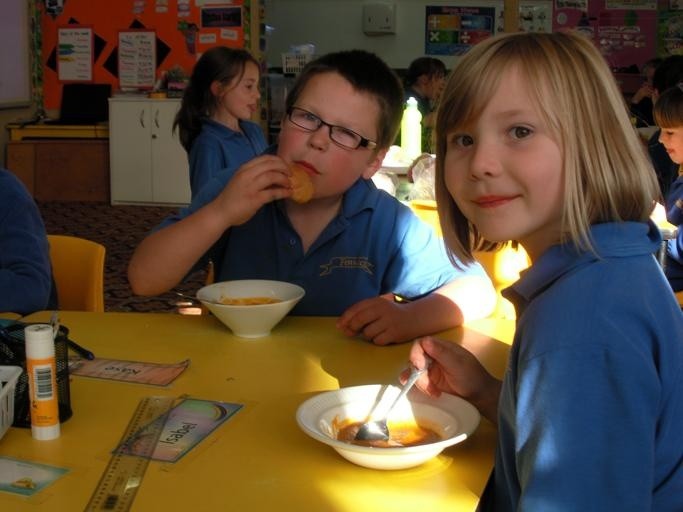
[0,0,33,110]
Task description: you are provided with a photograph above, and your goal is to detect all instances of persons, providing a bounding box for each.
[170,44,272,200]
[396,58,440,154]
[434,59,447,96]
[0,164,63,316]
[394,24,682,511]
[126,48,502,348]
[623,51,681,297]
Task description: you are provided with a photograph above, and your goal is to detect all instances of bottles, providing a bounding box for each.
[400,97,421,167]
[23,325,61,440]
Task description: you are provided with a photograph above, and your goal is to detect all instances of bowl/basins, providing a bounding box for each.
[195,280,306,340]
[638,127,658,140]
[295,383,483,471]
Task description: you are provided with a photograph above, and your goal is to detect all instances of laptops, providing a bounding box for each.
[44,83,111,124]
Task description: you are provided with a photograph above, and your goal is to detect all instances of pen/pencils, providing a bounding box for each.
[67,338,94,360]
[16,314,62,425]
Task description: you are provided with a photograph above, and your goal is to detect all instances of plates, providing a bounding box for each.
[378,167,409,175]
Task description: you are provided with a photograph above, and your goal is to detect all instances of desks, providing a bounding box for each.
[7,112,109,142]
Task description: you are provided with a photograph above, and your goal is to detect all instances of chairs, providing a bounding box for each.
[413,198,531,287]
[45,234,106,313]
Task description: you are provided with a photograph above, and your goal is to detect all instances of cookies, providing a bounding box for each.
[282,164,315,204]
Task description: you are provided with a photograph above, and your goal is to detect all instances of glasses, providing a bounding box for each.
[286,106,378,151]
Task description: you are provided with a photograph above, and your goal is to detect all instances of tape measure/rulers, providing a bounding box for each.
[83,396,176,512]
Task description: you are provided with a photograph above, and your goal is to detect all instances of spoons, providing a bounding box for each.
[350,356,434,447]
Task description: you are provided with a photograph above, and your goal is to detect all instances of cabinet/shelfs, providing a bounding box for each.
[108,94,193,209]
